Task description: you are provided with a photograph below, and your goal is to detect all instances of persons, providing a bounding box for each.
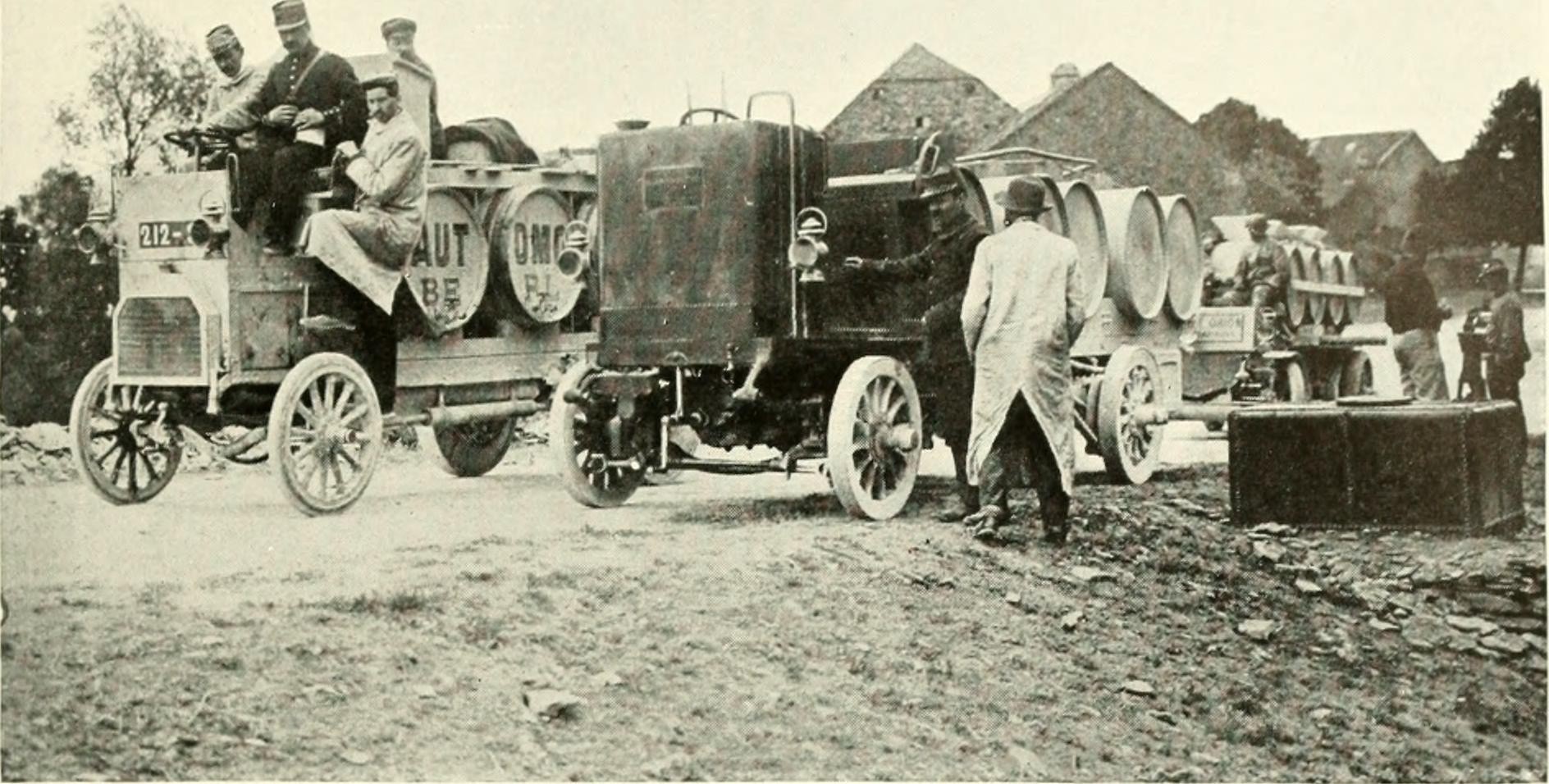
[1383,224,1453,401]
[230,0,365,255]
[1477,259,1526,402]
[177,23,264,184]
[840,168,988,522]
[1222,213,1290,331]
[295,75,429,414]
[961,180,1084,542]
[380,18,449,164]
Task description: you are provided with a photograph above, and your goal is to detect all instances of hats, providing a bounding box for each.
[993,177,1053,212]
[919,170,957,198]
[206,25,238,58]
[272,1,307,31]
[1477,259,1509,279]
[1246,214,1267,227]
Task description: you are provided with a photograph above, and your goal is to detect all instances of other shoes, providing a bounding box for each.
[930,500,968,523]
[300,314,357,333]
[382,412,395,421]
[262,242,288,256]
[974,516,997,539]
[1045,525,1068,542]
[962,504,1009,526]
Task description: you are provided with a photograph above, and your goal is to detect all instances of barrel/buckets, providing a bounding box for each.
[1096,185,1169,320]
[1321,251,1346,325]
[1160,195,1203,322]
[1304,245,1326,325]
[977,173,1070,240]
[1338,252,1362,322]
[395,184,489,333]
[1211,241,1306,328]
[1057,182,1108,320]
[471,186,581,324]
[571,200,600,313]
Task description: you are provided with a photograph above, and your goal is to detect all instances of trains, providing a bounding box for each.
[541,89,1207,524]
[1179,211,1391,431]
[65,108,597,522]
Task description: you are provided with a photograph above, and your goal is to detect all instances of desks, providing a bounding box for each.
[1226,398,1527,537]
